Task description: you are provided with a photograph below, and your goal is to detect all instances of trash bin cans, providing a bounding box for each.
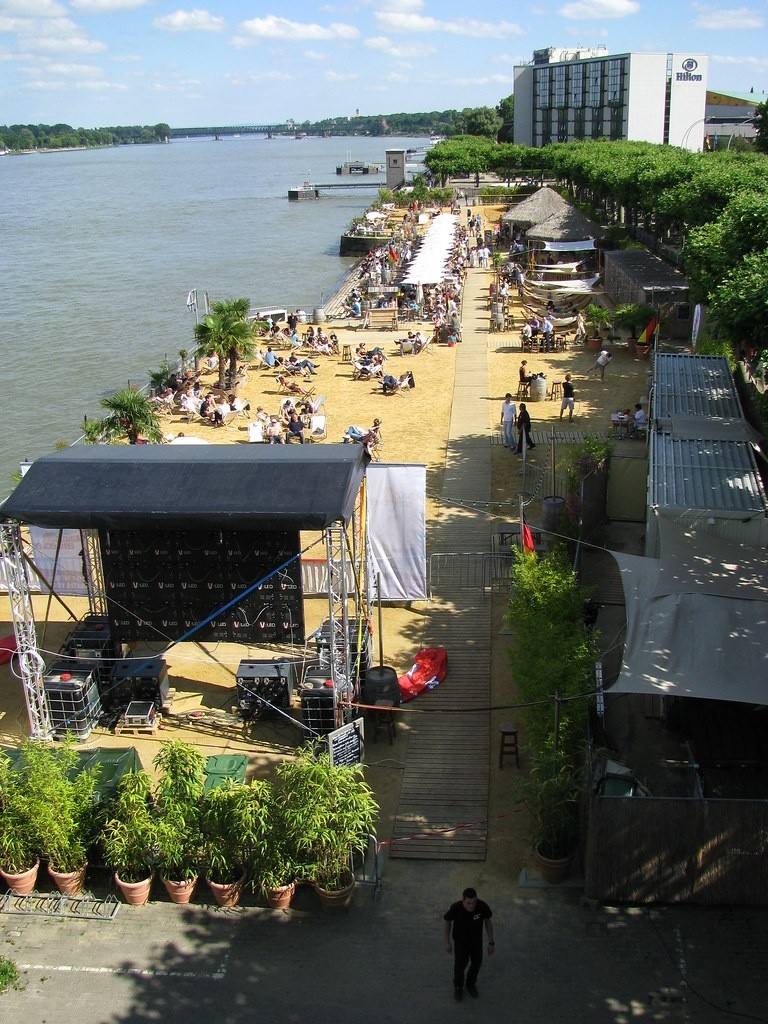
[203,753,250,792]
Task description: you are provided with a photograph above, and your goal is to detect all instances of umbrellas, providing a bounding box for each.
[500,188,606,242]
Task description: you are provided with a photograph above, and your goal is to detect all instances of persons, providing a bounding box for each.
[513,403,536,455]
[519,360,532,393]
[488,228,588,352]
[147,200,491,461]
[442,888,494,1002]
[627,403,647,437]
[584,350,612,384]
[500,393,518,451]
[559,373,576,422]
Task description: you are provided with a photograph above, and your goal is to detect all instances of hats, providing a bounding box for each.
[271,419,277,423]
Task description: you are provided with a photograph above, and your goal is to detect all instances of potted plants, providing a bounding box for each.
[0,733,379,910]
[526,718,595,886]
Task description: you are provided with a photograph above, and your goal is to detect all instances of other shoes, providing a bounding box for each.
[528,445,535,449]
[514,451,522,454]
[465,983,479,998]
[454,983,464,1001]
[569,419,574,423]
[378,381,384,384]
[511,445,516,450]
[343,434,350,438]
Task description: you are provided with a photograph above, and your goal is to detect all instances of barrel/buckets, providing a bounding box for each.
[493,313,504,330]
[381,270,391,283]
[313,309,324,325]
[361,301,370,317]
[490,284,497,296]
[542,496,566,533]
[491,303,502,317]
[365,666,399,714]
[530,378,546,401]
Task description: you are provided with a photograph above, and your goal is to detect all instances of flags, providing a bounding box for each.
[522,511,535,554]
[636,314,659,345]
[389,245,397,264]
[706,132,711,149]
[714,132,718,144]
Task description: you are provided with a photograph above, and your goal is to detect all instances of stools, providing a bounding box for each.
[498,721,519,770]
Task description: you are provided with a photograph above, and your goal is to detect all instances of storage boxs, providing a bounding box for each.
[124,701,155,726]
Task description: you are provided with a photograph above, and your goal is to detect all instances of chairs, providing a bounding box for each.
[162,202,649,458]
[372,699,398,746]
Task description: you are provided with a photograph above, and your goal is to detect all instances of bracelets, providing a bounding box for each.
[488,942,494,945]
